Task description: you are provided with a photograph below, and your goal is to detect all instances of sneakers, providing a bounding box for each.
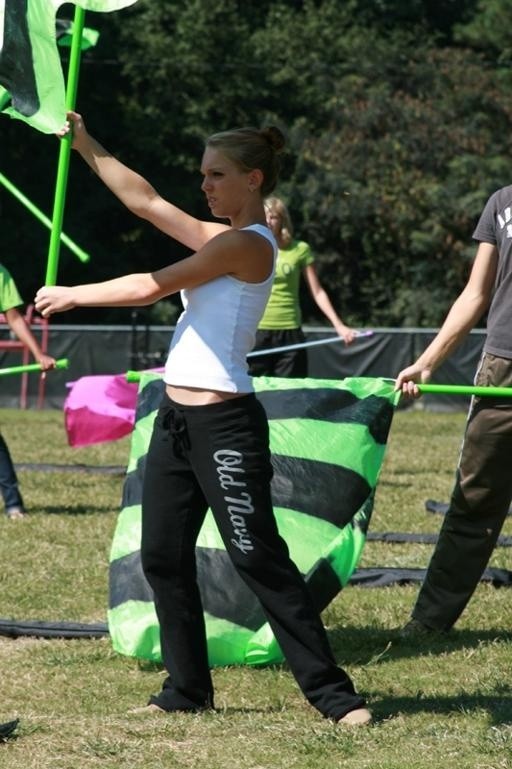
[338,707,372,728]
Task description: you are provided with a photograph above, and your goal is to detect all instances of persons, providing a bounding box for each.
[367,183,512,644]
[0,263,56,523]
[31,112,375,726]
[246,197,358,376]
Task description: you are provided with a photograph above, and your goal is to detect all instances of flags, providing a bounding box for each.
[106,370,401,668]
[0,0,139,136]
[63,367,166,447]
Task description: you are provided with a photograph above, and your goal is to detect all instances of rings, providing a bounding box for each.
[44,298,51,306]
